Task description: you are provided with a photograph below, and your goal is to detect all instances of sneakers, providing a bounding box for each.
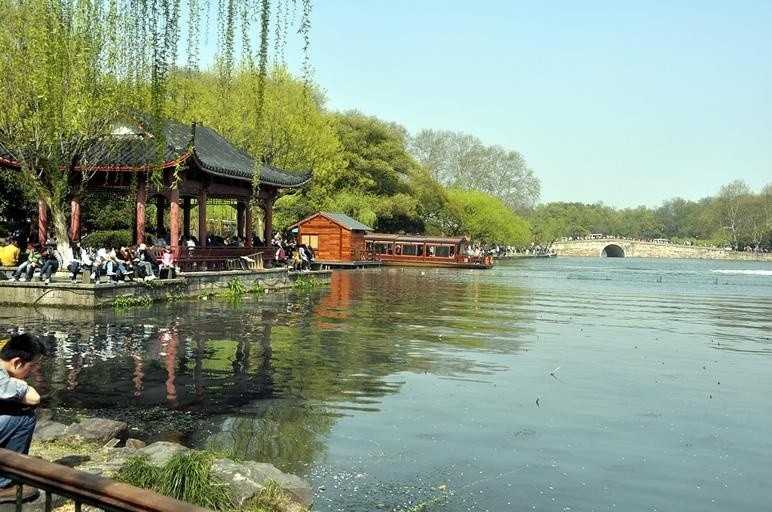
[9,271,157,285]
[0,483,39,502]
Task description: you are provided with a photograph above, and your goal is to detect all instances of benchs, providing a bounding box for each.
[146,244,275,271]
[0,266,93,284]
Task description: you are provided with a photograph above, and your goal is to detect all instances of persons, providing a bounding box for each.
[178,225,316,271]
[473,245,479,258]
[0,333,46,504]
[449,252,454,259]
[0,223,174,284]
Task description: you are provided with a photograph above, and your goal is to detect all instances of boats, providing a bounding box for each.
[365,232,498,272]
